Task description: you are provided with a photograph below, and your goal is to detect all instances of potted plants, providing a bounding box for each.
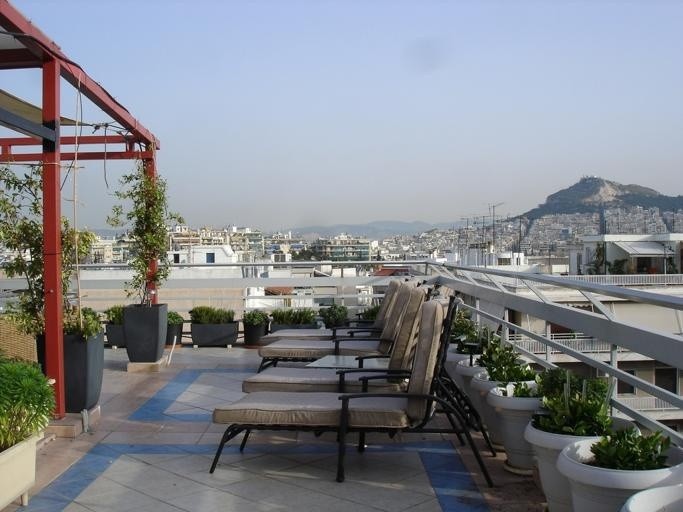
[0,356,56,512]
[102,304,125,347]
[167,311,184,345]
[0,160,105,413]
[188,302,379,346]
[105,156,186,362]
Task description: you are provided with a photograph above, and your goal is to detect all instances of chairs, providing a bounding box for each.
[209,296,460,483]
[260,279,401,368]
[256,284,415,372]
[241,285,434,437]
[0,318,40,364]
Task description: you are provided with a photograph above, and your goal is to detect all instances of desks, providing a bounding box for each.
[305,353,392,370]
